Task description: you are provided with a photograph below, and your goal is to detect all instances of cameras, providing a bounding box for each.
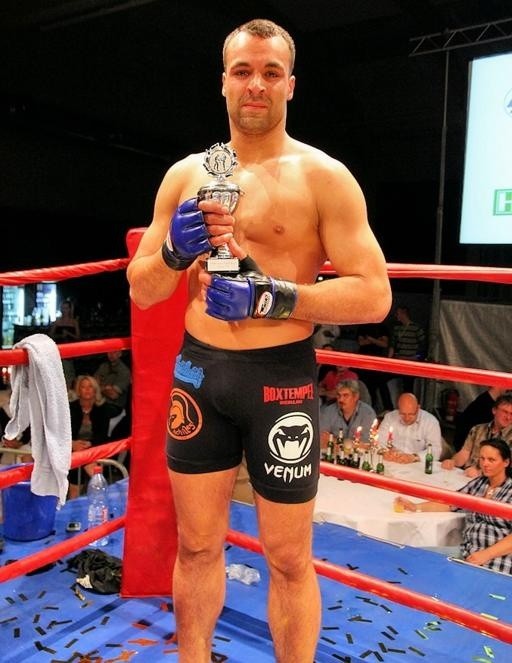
[67,521,81,531]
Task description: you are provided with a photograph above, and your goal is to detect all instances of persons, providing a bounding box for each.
[1,335,132,499]
[125,18,392,663]
[315,304,426,417]
[387,438,512,576]
[315,365,512,478]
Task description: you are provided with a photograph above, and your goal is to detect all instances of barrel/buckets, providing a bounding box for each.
[0,462,60,542]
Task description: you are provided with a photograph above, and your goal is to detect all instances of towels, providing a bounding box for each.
[4,333,72,506]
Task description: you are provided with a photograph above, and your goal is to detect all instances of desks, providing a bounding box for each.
[313,449,475,549]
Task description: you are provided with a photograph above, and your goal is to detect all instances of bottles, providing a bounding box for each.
[25,303,56,326]
[424,443,432,473]
[324,427,384,475]
[2,287,21,344]
[87,466,108,545]
[36,291,54,306]
[393,490,404,512]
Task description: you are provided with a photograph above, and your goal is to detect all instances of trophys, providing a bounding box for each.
[197,140,245,273]
